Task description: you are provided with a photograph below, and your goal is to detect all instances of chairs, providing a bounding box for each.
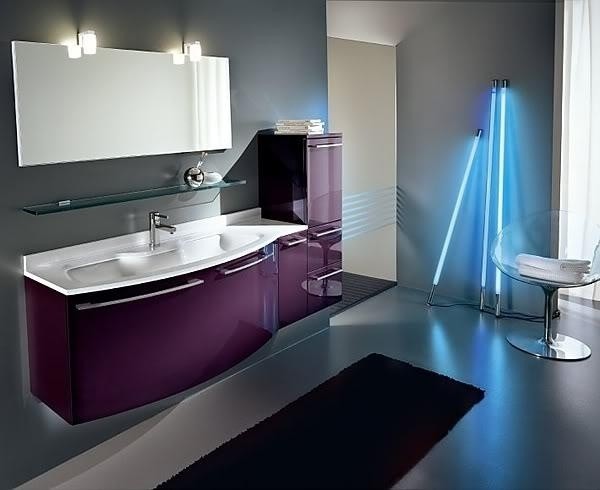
[491,209,600,362]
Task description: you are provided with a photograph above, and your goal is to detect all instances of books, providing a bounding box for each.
[274,119,325,135]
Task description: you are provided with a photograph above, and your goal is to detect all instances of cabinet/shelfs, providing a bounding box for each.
[24,132,344,426]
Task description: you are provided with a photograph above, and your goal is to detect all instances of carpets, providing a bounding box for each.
[150,354,485,490]
[330,271,398,320]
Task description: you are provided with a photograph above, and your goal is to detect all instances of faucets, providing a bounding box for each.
[148,210,177,245]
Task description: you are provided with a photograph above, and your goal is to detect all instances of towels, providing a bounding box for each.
[516,253,592,284]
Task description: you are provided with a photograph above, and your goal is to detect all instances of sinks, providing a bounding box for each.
[63,230,265,284]
